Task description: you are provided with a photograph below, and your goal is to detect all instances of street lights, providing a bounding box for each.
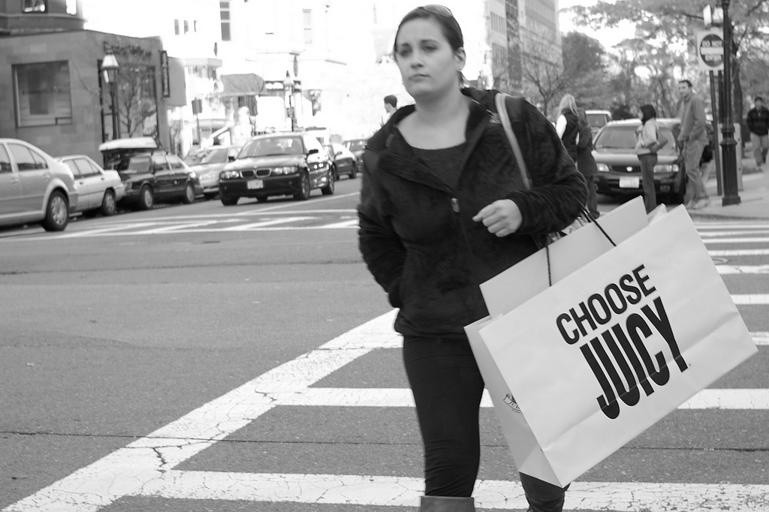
[280,67,297,132]
[701,4,727,197]
[99,48,123,141]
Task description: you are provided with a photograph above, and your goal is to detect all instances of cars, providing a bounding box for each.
[568,105,714,205]
[0,137,81,233]
[342,138,369,173]
[54,154,128,218]
[183,144,245,201]
[216,132,339,207]
[110,150,206,212]
[320,142,359,183]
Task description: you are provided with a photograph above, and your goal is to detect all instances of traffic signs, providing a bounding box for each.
[696,26,724,71]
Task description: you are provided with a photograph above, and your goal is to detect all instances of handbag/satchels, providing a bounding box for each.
[462,184,759,489]
[494,92,592,250]
[640,124,668,154]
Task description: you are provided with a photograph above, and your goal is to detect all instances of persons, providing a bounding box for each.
[359,4,588,511]
[635,103,660,214]
[382,94,400,117]
[746,96,769,173]
[558,94,579,163]
[575,118,599,221]
[677,79,711,211]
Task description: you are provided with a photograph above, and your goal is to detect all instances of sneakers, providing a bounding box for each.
[692,199,710,209]
